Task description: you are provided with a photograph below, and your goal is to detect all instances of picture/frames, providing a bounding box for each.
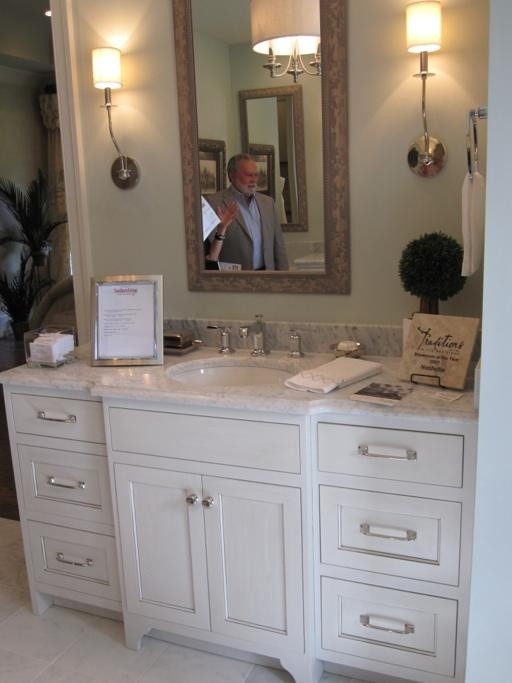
[248,144,275,197]
[198,138,228,196]
[90,274,164,367]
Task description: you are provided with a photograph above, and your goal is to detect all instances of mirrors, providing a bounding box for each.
[238,84,308,232]
[172,0,351,295]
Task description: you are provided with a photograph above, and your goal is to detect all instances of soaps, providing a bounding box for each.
[335,339,360,353]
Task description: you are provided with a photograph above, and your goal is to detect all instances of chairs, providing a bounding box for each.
[28,275,76,330]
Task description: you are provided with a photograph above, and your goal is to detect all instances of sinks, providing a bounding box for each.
[164,353,298,395]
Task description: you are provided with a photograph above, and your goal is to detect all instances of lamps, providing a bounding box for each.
[405,1,448,177]
[91,47,140,189]
[249,0,322,83]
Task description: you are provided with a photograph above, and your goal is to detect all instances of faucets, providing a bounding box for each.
[239,313,267,355]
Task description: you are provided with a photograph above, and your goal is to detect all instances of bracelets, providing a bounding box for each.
[215,233,226,241]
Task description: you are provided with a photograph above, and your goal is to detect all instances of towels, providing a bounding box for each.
[460,172,485,277]
[285,357,385,394]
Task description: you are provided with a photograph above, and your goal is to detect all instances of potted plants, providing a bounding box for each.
[0,245,56,341]
[397,229,468,353]
[0,168,68,267]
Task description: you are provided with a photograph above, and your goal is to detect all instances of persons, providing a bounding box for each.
[204,154,290,271]
[204,199,241,270]
